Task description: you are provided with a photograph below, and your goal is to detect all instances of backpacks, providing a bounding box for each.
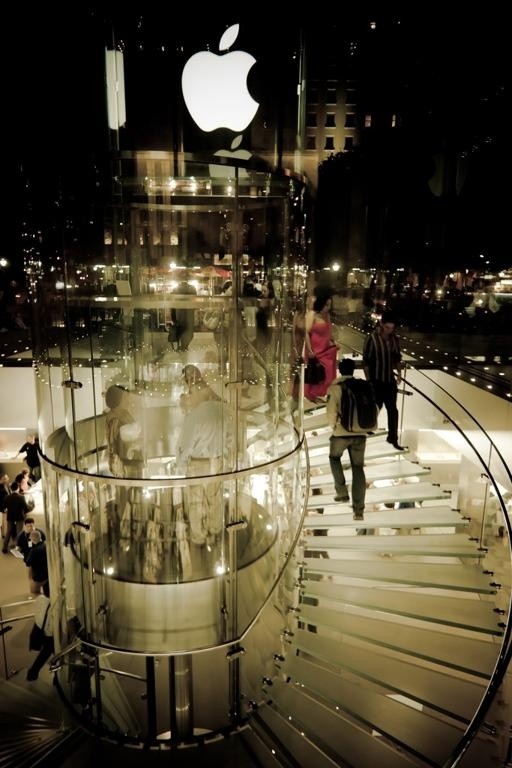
[327,377,378,433]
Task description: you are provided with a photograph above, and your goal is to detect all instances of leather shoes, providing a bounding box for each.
[387,436,404,449]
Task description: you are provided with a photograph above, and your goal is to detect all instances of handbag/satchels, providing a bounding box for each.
[29,622,45,650]
[304,356,325,384]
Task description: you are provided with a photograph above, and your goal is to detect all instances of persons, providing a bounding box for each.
[362,312,405,453]
[341,462,511,543]
[105,364,230,552]
[0,428,72,681]
[1,264,328,375]
[301,292,339,405]
[326,357,380,521]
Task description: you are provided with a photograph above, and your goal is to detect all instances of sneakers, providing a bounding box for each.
[335,495,349,501]
[353,512,363,519]
